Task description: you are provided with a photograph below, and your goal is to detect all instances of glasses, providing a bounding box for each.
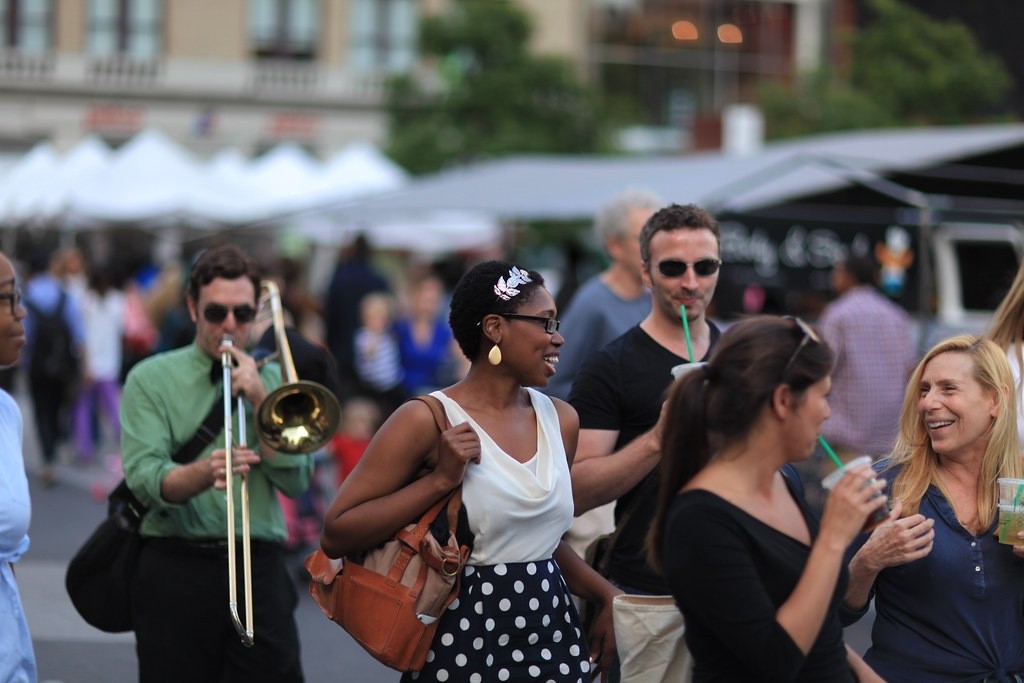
[476,311,561,334]
[781,314,820,376]
[0,287,24,314]
[202,301,258,324]
[651,258,722,278]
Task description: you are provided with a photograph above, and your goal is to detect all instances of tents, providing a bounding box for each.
[0,135,413,227]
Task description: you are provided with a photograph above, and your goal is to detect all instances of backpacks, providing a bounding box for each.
[24,288,83,404]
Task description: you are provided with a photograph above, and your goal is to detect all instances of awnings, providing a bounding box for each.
[316,123,1024,221]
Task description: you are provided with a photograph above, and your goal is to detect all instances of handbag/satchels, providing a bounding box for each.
[304,394,473,672]
[611,592,695,683]
[65,478,148,633]
[576,525,619,640]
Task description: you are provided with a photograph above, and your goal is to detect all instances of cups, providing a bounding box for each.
[996,478,1024,546]
[821,455,893,535]
[670,362,709,384]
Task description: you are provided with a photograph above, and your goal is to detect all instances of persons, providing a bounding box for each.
[322,262,626,683]
[0,251,38,683]
[647,314,888,683]
[19,233,503,549]
[120,245,311,683]
[842,265,1024,683]
[550,199,655,395]
[812,232,924,473]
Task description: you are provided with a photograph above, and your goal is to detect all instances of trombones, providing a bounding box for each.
[221,281,342,648]
